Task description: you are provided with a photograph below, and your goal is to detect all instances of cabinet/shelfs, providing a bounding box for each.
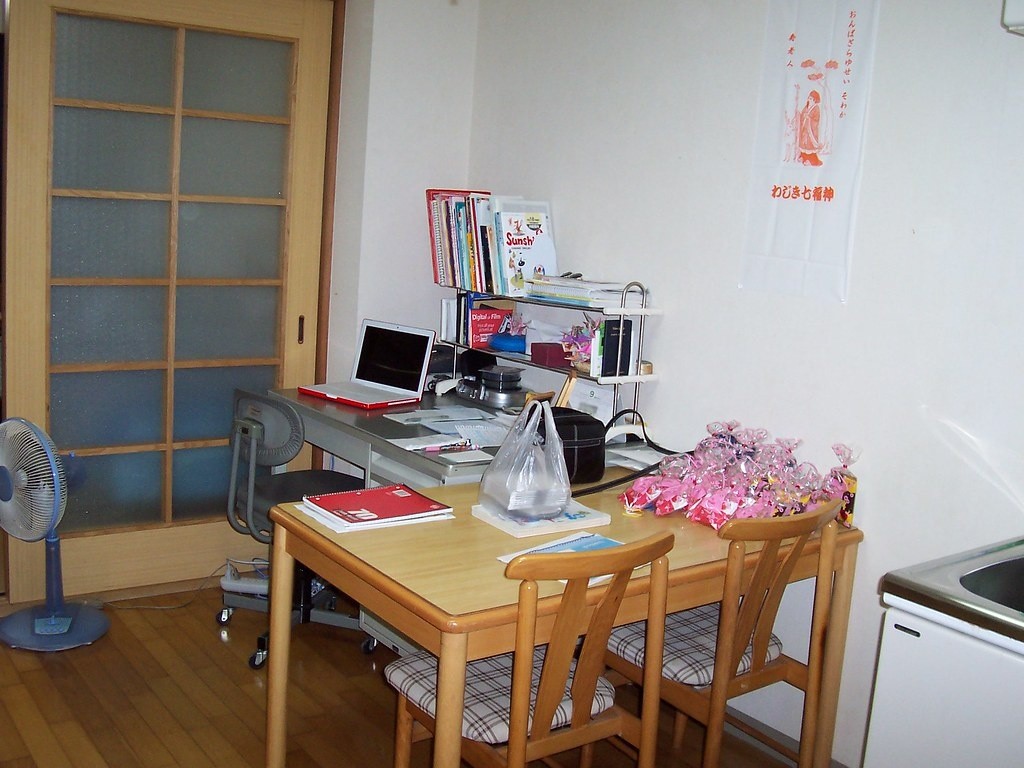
[436,280,664,445]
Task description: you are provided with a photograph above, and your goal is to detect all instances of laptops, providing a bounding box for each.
[297,319,436,411]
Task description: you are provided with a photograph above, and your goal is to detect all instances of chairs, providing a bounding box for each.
[582,498,844,768]
[384,529,675,768]
[218,387,379,667]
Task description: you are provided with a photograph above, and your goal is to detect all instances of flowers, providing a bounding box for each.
[557,317,603,362]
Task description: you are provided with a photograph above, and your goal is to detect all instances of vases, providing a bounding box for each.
[574,359,590,373]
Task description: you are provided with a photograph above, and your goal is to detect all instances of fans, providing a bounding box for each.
[0,416,111,652]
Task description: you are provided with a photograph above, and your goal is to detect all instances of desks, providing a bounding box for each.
[267,466,864,767]
[268,390,659,659]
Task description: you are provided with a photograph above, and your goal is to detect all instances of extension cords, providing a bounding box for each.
[219,575,269,596]
